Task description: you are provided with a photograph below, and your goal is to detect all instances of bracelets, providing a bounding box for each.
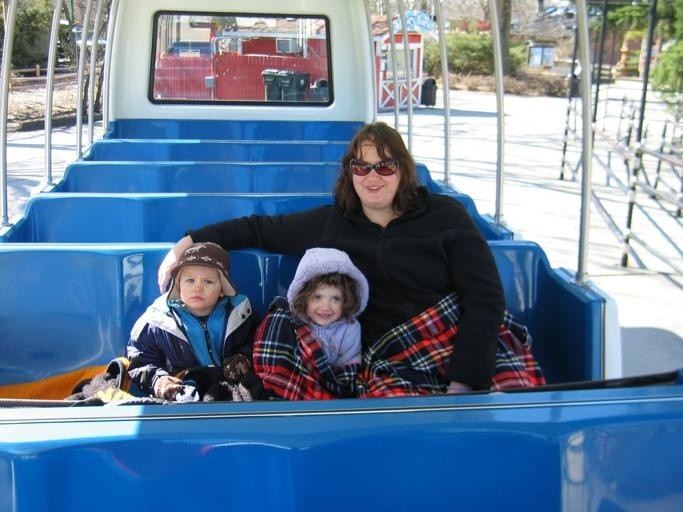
[446,385,473,391]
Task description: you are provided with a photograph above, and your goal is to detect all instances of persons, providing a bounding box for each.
[128,241,262,403]
[250,244,372,399]
[160,120,547,399]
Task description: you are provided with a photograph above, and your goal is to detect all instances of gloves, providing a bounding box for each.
[155,376,184,401]
[223,353,251,383]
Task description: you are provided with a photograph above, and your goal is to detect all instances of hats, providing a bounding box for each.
[167,242,236,300]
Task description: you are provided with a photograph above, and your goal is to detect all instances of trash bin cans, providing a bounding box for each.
[420,78,437,108]
[259,69,330,103]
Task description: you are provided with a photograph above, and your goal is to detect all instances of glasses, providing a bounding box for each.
[350,158,399,176]
